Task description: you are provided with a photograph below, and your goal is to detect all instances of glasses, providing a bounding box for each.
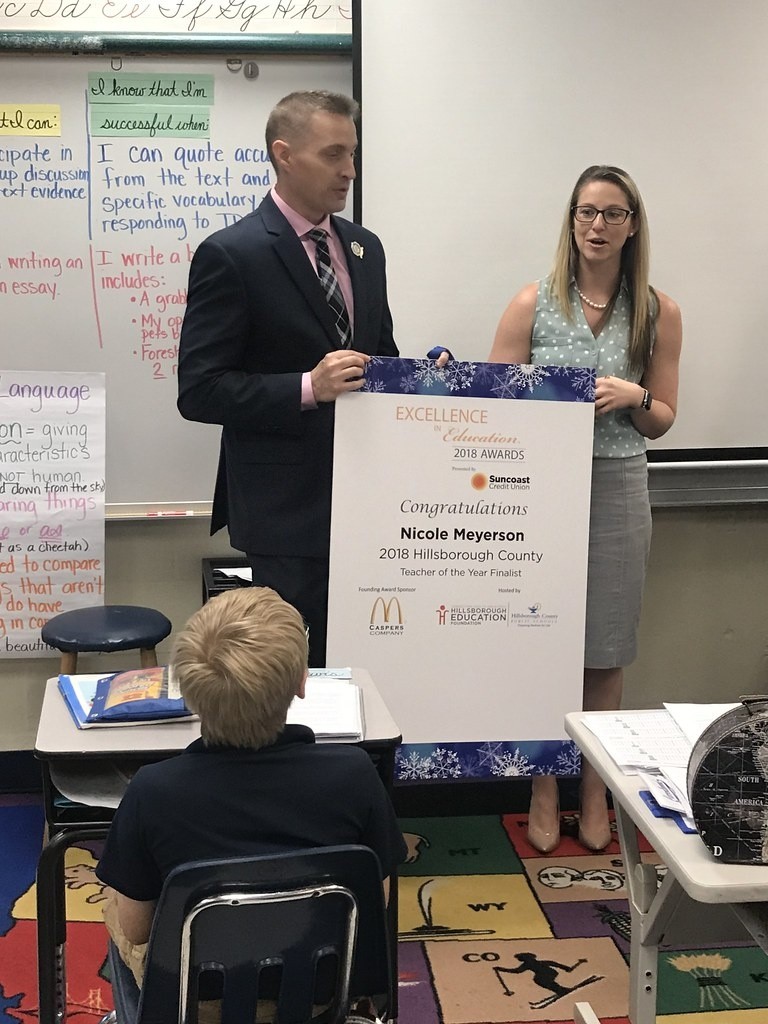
[570,204,636,225]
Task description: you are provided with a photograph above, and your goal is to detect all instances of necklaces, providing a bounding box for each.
[571,284,607,309]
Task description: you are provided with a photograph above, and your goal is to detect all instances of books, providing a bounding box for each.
[55,671,202,731]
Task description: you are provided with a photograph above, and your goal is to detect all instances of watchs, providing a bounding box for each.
[628,382,652,414]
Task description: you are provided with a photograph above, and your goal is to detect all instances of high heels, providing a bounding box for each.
[528,782,562,854]
[578,781,611,851]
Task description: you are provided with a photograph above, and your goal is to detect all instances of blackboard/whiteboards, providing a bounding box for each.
[0,53,354,521]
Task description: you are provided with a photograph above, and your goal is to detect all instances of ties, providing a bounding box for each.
[309,225,353,353]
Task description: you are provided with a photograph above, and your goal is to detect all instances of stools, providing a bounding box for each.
[43,605,172,671]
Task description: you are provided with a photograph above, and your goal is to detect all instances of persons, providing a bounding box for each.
[93,583,410,1024]
[427,164,683,852]
[176,90,399,668]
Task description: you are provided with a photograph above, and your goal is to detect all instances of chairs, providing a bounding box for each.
[107,845,397,1024]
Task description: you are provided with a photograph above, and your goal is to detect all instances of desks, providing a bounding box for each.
[33,664,402,1023]
[566,702,768,1023]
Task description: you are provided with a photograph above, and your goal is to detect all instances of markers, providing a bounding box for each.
[145,511,194,517]
